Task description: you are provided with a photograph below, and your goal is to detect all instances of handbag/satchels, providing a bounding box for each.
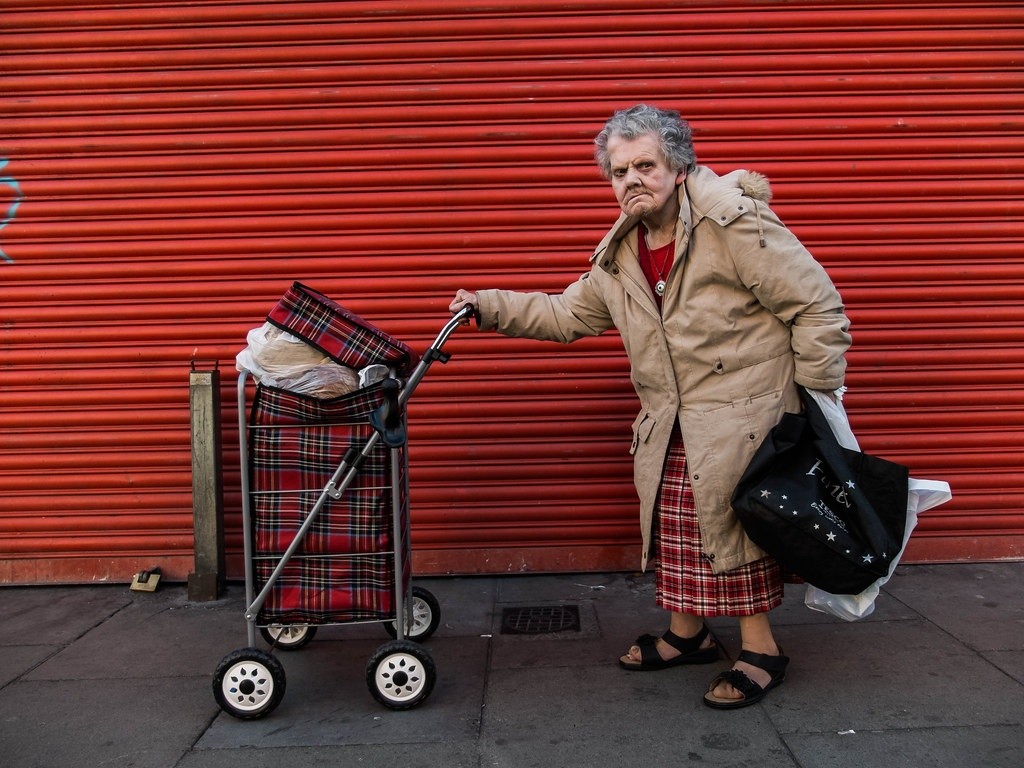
[804,385,952,621]
[730,381,910,595]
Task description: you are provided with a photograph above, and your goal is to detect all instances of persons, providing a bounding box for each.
[450,102,853,711]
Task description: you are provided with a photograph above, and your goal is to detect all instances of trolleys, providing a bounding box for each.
[212,304,477,726]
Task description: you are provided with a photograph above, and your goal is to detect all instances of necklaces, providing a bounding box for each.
[644,211,680,296]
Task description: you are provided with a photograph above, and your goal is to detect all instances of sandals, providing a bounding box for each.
[618,621,719,672]
[703,643,791,711]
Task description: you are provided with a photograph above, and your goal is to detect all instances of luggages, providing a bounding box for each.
[212,283,473,721]
